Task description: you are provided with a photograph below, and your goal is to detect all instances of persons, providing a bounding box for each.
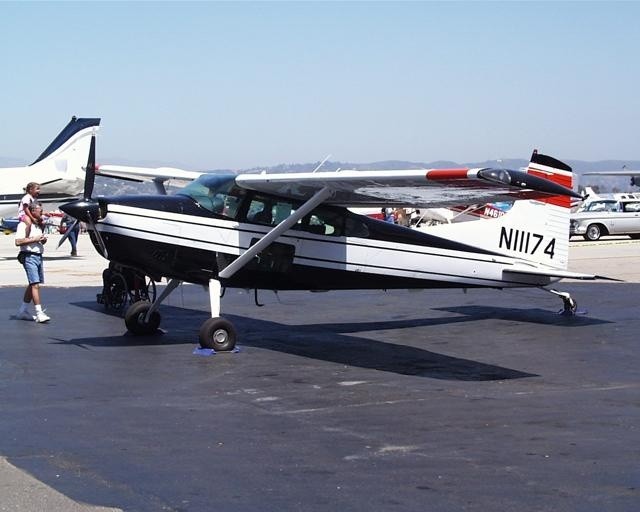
[411,210,423,226]
[13,204,51,322]
[380,207,409,228]
[59,213,81,255]
[17,183,47,238]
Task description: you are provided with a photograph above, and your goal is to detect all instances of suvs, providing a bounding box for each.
[569,199,640,241]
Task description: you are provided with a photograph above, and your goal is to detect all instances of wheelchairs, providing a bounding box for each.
[95,261,160,316]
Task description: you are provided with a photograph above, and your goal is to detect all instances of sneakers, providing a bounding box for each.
[16,311,37,320]
[35,313,51,323]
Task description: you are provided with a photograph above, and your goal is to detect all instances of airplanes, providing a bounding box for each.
[64,143,629,354]
[0,115,102,204]
